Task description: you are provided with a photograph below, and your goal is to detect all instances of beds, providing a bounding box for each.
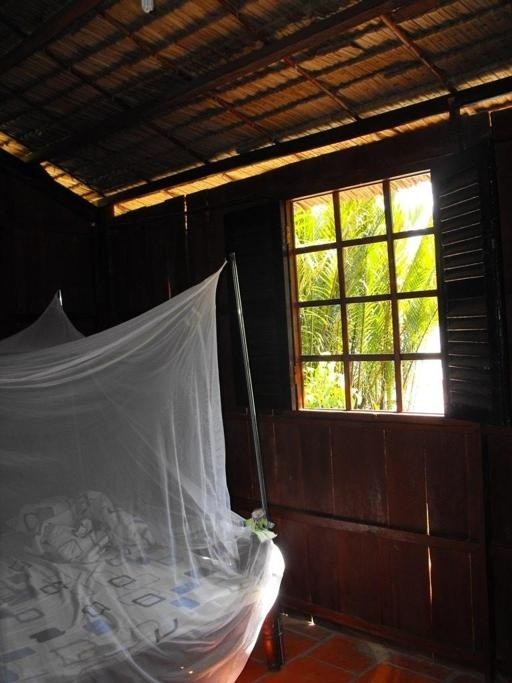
[1,251,285,683]
